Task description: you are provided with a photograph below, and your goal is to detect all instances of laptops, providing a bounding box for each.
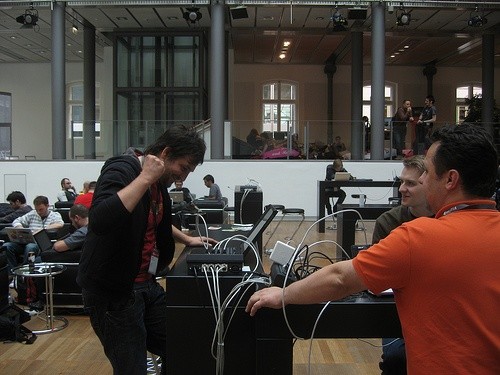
[189,203,277,259]
[32,230,56,254]
[168,190,184,204]
[333,171,351,181]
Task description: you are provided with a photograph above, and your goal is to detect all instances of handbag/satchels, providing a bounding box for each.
[0,305,33,342]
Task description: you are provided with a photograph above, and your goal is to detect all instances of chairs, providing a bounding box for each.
[183,196,228,229]
[0,199,86,315]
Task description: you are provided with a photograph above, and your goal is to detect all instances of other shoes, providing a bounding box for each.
[333,207,337,218]
[9,280,15,287]
[327,207,332,214]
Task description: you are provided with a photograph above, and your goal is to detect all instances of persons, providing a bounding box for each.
[29,206,90,311]
[2,195,64,290]
[0,191,33,225]
[417,95,436,145]
[75,126,221,375]
[391,100,414,156]
[369,155,436,375]
[203,174,223,202]
[247,127,346,160]
[326,159,354,217]
[170,179,193,229]
[58,178,96,209]
[242,124,500,375]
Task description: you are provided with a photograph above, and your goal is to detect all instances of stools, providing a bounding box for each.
[264,204,305,255]
[224,206,235,224]
[389,197,401,204]
[351,194,367,231]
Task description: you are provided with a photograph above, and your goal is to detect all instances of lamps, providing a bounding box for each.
[395,8,412,26]
[347,4,368,20]
[15,3,39,29]
[330,5,348,33]
[183,6,202,23]
[467,4,488,28]
[230,3,249,20]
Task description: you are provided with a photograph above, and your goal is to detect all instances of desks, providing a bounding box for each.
[336,203,398,262]
[161,223,264,375]
[10,264,69,335]
[317,180,402,233]
[256,277,404,375]
